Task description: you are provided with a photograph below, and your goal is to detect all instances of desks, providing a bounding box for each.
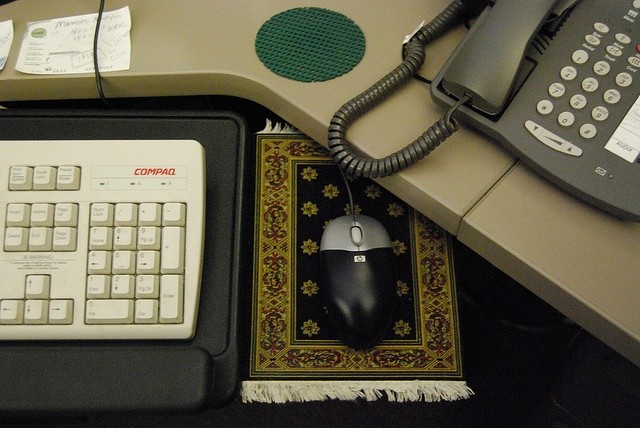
[0,1,640,365]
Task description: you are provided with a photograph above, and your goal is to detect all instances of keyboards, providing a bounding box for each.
[0,138,210,341]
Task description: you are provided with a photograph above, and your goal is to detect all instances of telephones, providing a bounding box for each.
[328,0,639,222]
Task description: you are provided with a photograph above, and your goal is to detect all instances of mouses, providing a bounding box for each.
[318,214,400,349]
